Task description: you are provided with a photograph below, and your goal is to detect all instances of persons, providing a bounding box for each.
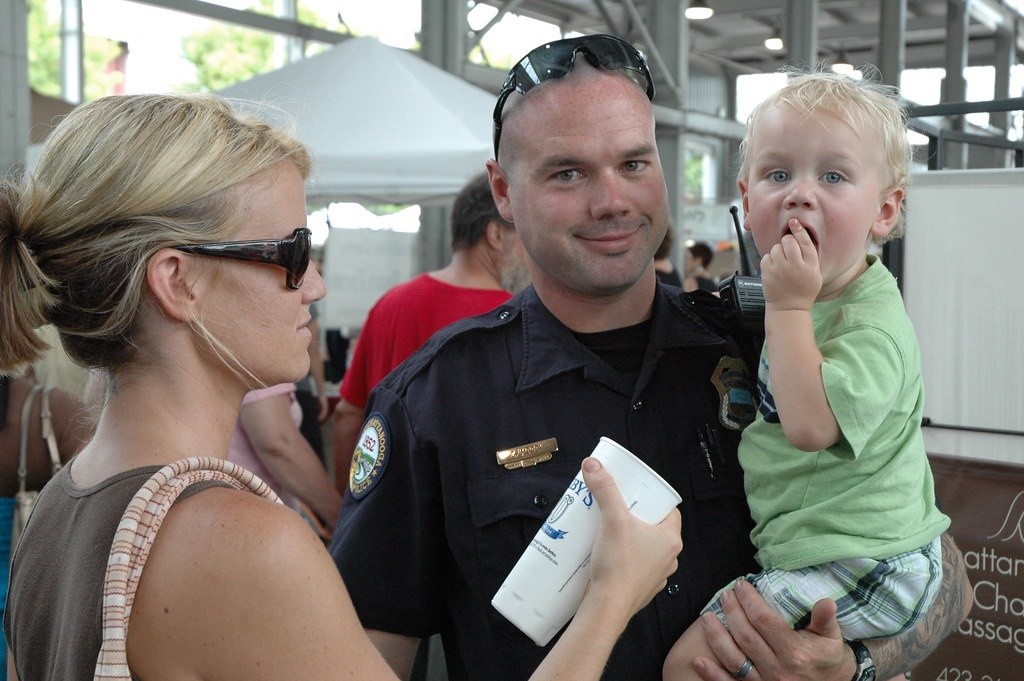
[0,94,682,681]
[335,174,526,493]
[329,46,973,681]
[654,225,717,295]
[224,304,341,529]
[105,42,130,93]
[662,74,951,681]
[0,376,95,681]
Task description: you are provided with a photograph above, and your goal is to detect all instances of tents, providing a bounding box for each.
[28,35,500,202]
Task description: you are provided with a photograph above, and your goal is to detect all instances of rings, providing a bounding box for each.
[733,658,753,678]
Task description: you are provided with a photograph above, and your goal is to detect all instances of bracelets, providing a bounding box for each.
[846,641,876,681]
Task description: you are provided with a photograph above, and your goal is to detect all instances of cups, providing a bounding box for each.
[490,435,682,648]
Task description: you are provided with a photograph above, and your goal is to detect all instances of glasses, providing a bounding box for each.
[493,34,656,163]
[146,228,312,290]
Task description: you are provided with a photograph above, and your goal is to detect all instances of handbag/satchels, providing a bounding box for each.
[10,382,62,555]
